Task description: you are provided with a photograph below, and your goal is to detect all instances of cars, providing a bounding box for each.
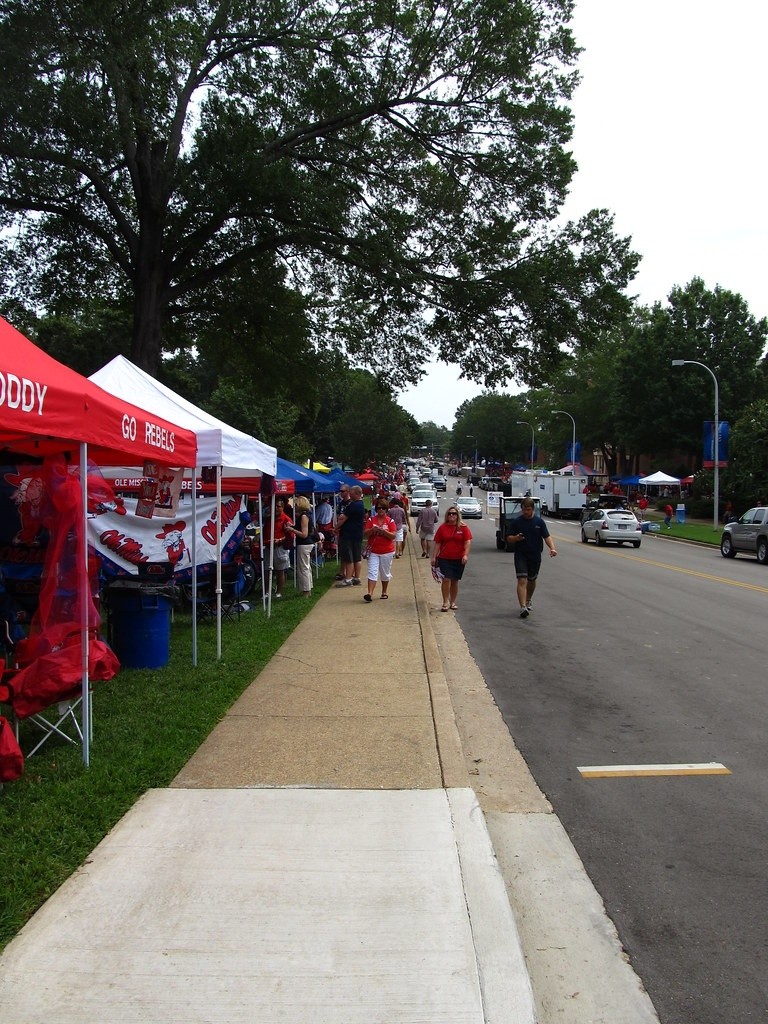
[580,494,630,526]
[398,455,512,498]
[452,496,484,519]
[581,508,643,548]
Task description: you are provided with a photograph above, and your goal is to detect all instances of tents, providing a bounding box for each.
[638,471,681,497]
[277,457,339,579]
[306,462,372,529]
[616,475,651,496]
[104,475,295,610]
[62,355,277,660]
[0,314,197,765]
[680,476,695,494]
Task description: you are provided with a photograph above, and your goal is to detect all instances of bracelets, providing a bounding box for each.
[334,531,337,533]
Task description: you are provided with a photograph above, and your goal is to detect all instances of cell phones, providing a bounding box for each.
[520,533,524,538]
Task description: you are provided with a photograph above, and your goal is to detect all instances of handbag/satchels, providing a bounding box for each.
[299,513,321,545]
[282,531,295,551]
[361,543,372,559]
[432,556,440,569]
[402,523,410,533]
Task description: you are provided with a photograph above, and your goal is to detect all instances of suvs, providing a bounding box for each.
[720,506,768,565]
[408,489,441,516]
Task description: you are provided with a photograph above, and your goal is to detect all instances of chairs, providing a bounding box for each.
[9,632,97,763]
[181,580,240,629]
[309,545,323,571]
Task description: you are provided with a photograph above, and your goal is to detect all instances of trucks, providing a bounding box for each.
[507,469,588,520]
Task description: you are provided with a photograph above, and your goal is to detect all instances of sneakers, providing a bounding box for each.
[519,607,529,618]
[352,578,362,586]
[525,601,534,611]
[335,579,354,588]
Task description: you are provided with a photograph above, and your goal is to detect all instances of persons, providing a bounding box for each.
[722,501,733,525]
[416,500,438,558]
[582,479,688,530]
[456,481,464,489]
[470,481,473,497]
[430,507,472,612]
[283,496,314,599]
[314,499,332,525]
[420,473,423,479]
[260,501,292,600]
[444,474,446,479]
[506,499,557,618]
[332,465,411,601]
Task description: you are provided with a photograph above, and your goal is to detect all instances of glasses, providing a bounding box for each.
[376,507,387,510]
[447,512,458,515]
[339,490,347,493]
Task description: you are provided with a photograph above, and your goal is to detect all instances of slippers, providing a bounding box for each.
[450,604,458,609]
[380,594,389,599]
[441,605,449,612]
[363,593,373,602]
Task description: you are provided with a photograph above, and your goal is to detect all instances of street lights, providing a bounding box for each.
[551,410,575,476]
[672,360,719,532]
[466,435,478,473]
[516,421,535,469]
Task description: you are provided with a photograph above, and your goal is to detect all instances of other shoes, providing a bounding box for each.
[395,552,402,559]
[275,593,282,600]
[667,526,671,530]
[426,555,430,558]
[421,552,427,557]
[260,593,269,600]
[334,573,354,581]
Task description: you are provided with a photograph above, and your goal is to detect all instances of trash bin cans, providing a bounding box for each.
[104,578,178,670]
[676,509,686,523]
